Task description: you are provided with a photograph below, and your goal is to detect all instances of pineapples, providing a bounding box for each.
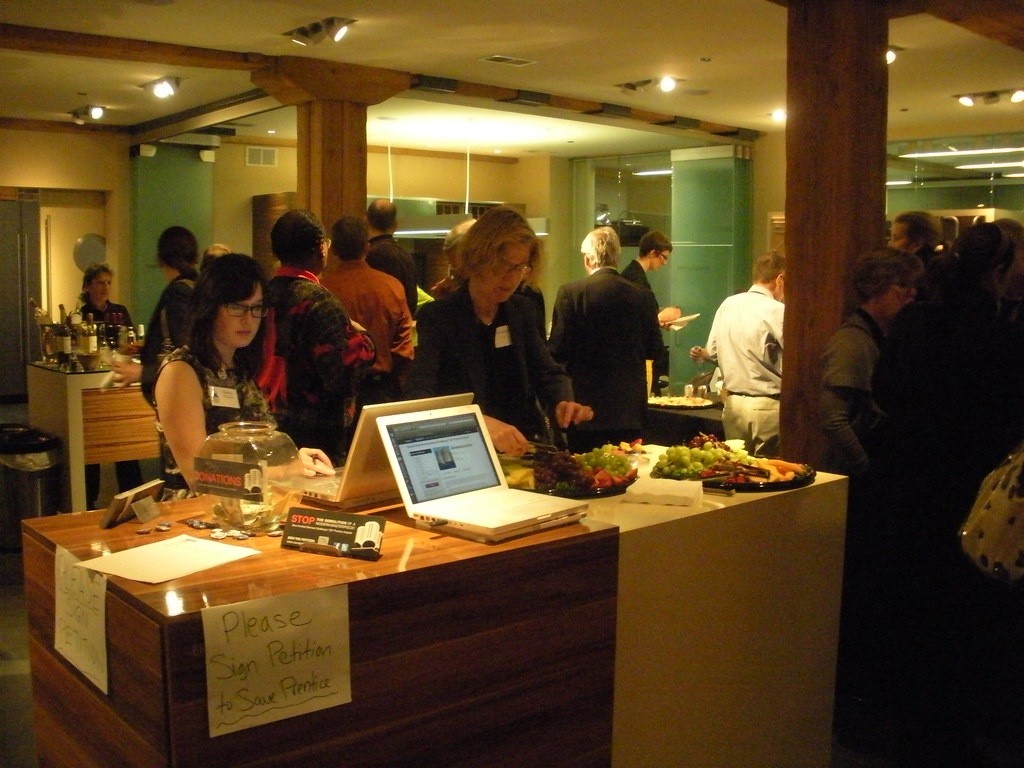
[653,435,736,478]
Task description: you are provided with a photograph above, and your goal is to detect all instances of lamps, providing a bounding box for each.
[162,77,180,96]
[385,127,473,234]
[290,24,313,49]
[72,108,88,125]
[984,93,1000,106]
[85,105,104,120]
[143,81,167,99]
[1008,89,1024,103]
[958,94,976,106]
[321,17,348,43]
[464,141,551,236]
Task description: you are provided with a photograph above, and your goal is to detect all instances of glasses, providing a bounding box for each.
[222,303,267,318]
[322,236,332,248]
[655,249,668,260]
[499,260,534,275]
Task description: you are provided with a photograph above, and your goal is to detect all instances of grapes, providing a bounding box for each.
[531,448,633,495]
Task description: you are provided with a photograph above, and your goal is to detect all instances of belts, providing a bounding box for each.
[725,388,781,401]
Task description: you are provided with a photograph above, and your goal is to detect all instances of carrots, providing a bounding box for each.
[743,459,808,483]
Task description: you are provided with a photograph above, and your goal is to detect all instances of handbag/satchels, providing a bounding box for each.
[959,444,1023,588]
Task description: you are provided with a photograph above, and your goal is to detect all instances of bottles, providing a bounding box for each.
[43,303,145,365]
[684,384,693,397]
[698,385,707,397]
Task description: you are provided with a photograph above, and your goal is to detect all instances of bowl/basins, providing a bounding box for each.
[75,353,103,370]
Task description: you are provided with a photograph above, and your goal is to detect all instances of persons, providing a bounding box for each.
[78,265,144,510]
[708,252,785,461]
[141,200,479,472]
[823,210,1024,768]
[414,204,593,459]
[154,253,336,501]
[622,231,681,397]
[547,227,660,455]
[690,346,723,394]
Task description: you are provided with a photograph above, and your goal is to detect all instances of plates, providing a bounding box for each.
[519,470,639,496]
[649,401,718,409]
[703,454,817,490]
[666,313,700,325]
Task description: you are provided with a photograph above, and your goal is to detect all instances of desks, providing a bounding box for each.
[646,403,726,447]
[24,358,161,514]
[21,466,621,768]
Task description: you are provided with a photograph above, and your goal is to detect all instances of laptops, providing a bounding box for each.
[301,392,475,502]
[376,403,589,536]
